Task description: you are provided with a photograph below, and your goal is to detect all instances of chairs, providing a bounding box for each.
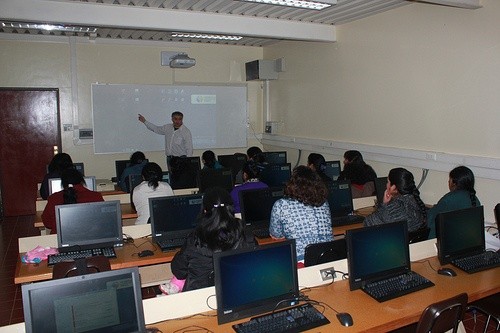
[414,292,469,333]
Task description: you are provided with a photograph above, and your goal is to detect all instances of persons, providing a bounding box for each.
[309,149,378,217]
[156,189,258,298]
[202,146,271,219]
[121,151,173,225]
[270,165,335,269]
[363,168,427,233]
[138,111,193,165]
[426,166,481,239]
[40,153,105,234]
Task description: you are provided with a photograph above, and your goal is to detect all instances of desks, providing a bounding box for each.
[148,245,500,332]
[33,180,136,227]
[14,198,433,286]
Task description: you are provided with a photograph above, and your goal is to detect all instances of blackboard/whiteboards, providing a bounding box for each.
[90,82,248,154]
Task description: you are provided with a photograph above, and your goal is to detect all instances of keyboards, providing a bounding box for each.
[156,237,186,252]
[231,302,330,333]
[48,246,118,266]
[360,271,436,304]
[250,230,272,239]
[450,251,500,274]
[332,214,366,227]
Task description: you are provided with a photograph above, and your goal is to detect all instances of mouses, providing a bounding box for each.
[138,250,154,258]
[437,268,457,277]
[336,311,353,327]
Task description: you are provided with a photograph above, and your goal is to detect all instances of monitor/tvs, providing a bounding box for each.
[115,150,389,225]
[346,219,410,291]
[48,177,95,195]
[147,193,203,237]
[54,200,122,251]
[20,266,147,333]
[46,162,84,178]
[213,239,300,326]
[435,205,485,266]
